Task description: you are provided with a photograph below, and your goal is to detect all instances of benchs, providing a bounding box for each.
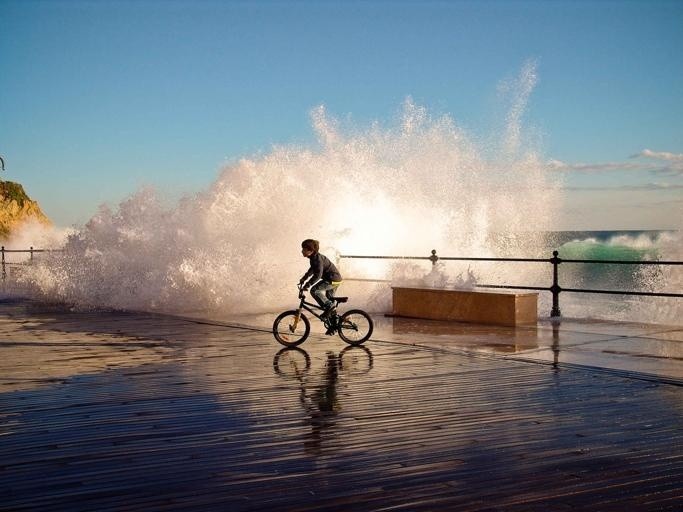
[382,284,540,331]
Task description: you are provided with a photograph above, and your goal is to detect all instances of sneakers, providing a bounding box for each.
[320,303,337,319]
[325,322,340,335]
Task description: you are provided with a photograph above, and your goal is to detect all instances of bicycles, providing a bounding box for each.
[272,281,373,347]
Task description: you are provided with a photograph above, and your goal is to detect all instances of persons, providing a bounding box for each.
[298,234,344,322]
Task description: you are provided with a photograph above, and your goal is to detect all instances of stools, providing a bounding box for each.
[330,295,348,307]
